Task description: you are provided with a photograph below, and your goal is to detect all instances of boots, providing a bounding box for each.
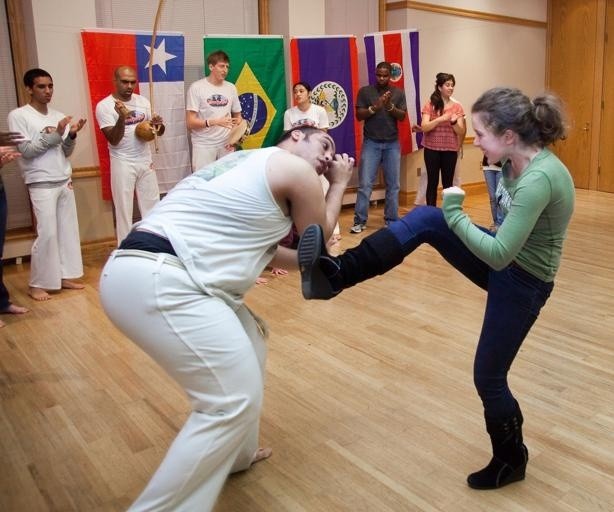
[467,400,529,490]
[298,224,404,300]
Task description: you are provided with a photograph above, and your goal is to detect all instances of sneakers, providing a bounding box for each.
[351,224,367,233]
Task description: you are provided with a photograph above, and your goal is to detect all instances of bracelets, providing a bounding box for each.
[450,122,458,125]
[385,104,394,111]
[368,106,374,115]
[205,120,209,127]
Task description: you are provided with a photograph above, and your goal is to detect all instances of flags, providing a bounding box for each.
[203,34,286,153]
[364,29,423,158]
[81,28,192,201]
[287,36,363,169]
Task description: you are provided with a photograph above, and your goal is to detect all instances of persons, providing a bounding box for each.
[482,154,508,233]
[412,95,462,204]
[282,83,341,241]
[420,73,468,207]
[8,67,86,302]
[97,126,356,512]
[254,260,290,285]
[298,87,575,488]
[350,63,407,233]
[0,130,30,329]
[95,65,164,247]
